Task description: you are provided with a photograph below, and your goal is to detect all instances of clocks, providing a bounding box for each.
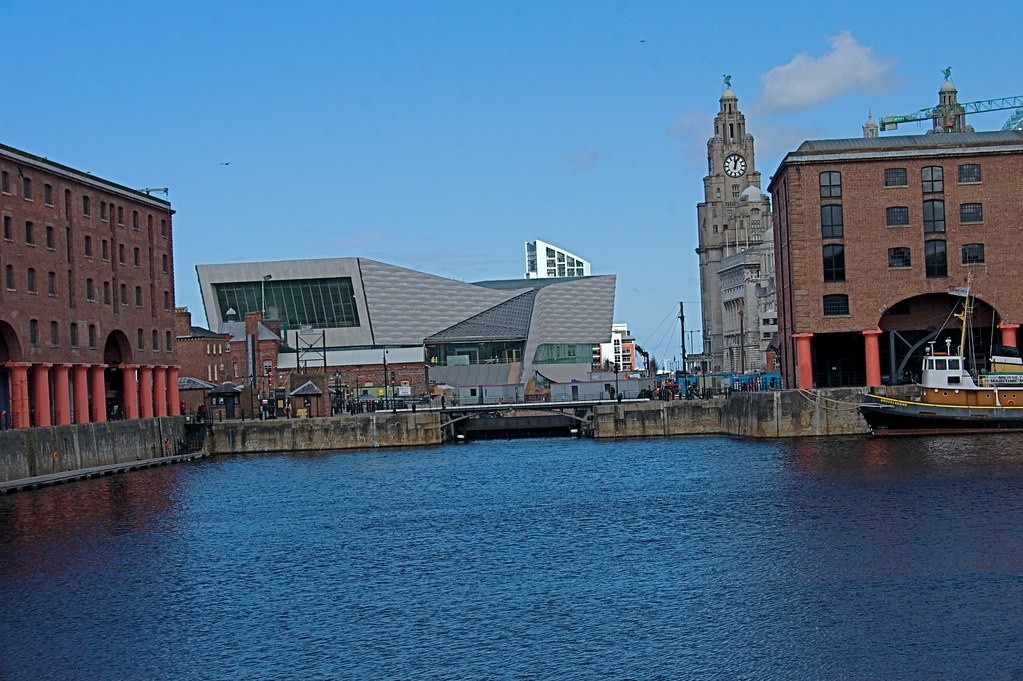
[723,154,747,178]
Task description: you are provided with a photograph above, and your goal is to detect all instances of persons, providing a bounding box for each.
[609,384,616,401]
[108,404,126,421]
[180,399,208,422]
[440,393,446,409]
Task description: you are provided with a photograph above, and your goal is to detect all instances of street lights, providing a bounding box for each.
[262,274,272,319]
[333,370,343,415]
[248,374,255,421]
[240,375,244,421]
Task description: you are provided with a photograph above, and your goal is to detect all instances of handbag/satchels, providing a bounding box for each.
[165,441,167,443]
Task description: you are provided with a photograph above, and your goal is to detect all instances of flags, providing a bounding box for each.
[945,284,970,297]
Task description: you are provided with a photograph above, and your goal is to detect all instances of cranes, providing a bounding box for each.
[880,95,1022,132]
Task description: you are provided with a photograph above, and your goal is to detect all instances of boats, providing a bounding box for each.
[859,273,1022,440]
